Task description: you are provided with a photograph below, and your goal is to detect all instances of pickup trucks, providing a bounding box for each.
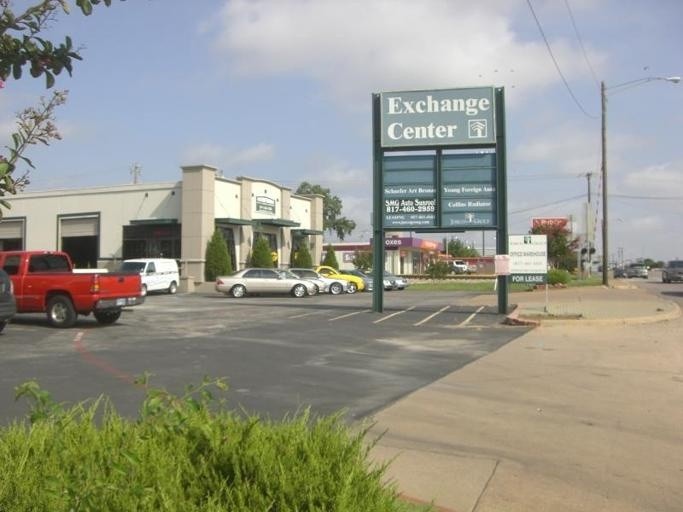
[0,250,145,329]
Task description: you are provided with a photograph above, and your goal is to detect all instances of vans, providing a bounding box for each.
[120,258,180,297]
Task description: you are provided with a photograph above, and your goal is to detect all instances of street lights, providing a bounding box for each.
[599,74,681,289]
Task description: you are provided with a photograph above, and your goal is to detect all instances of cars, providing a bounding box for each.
[214,267,315,298]
[625,259,683,283]
[287,265,408,295]
